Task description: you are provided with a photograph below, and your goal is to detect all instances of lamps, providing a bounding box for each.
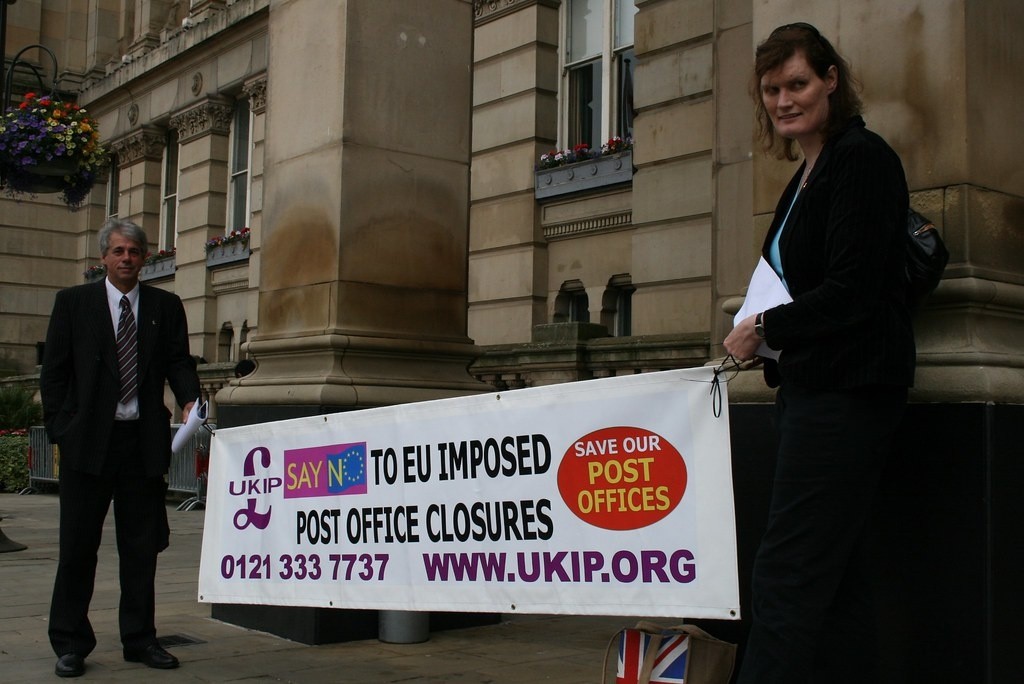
[181,16,193,28]
[122,55,132,64]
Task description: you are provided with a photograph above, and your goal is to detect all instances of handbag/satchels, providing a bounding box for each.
[601,621,738,684]
[902,208,949,320]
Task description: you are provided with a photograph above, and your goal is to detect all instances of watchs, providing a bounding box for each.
[754,313,765,337]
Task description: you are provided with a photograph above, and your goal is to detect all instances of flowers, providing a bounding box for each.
[82,265,106,279]
[143,247,176,263]
[204,226,250,253]
[0,92,119,216]
[535,135,632,172]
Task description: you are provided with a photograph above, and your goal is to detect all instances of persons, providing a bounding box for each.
[722,22,917,684]
[40,219,209,677]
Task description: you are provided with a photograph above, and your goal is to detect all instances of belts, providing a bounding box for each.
[114,420,139,427]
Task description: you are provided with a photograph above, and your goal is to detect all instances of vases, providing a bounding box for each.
[87,274,106,283]
[206,243,249,271]
[13,158,75,193]
[534,150,632,202]
[140,256,176,284]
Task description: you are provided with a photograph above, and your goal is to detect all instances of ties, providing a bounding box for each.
[116,295,138,406]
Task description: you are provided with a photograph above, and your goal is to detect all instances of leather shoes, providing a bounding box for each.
[123,644,179,669]
[55,652,85,677]
